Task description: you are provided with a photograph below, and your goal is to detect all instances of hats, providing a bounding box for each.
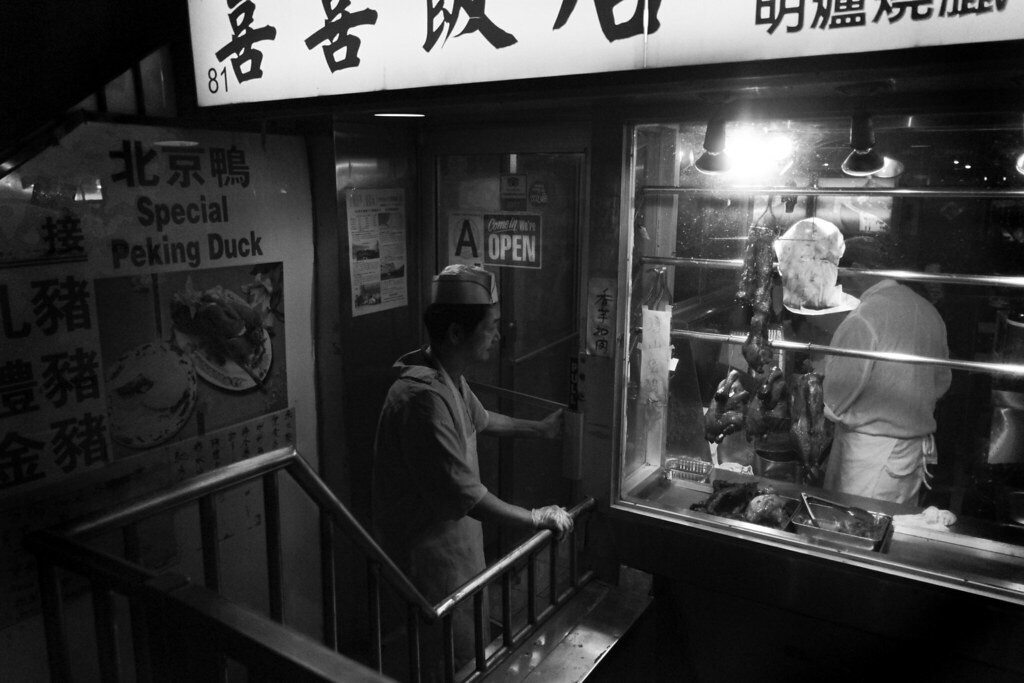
[430,262,499,306]
[837,200,889,237]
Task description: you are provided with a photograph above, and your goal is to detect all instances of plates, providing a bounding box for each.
[106,344,196,449]
[176,289,271,392]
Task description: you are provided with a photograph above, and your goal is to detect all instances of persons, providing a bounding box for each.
[822,236,978,507]
[368,263,574,683]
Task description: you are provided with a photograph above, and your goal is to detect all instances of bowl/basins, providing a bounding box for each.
[791,502,889,550]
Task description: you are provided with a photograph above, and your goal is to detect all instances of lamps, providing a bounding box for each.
[840,112,885,176]
[695,119,730,174]
[372,92,428,118]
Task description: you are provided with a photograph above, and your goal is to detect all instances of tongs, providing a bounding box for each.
[802,492,874,529]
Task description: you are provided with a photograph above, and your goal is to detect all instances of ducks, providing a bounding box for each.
[689,477,798,531]
[702,225,835,469]
[170,273,273,366]
